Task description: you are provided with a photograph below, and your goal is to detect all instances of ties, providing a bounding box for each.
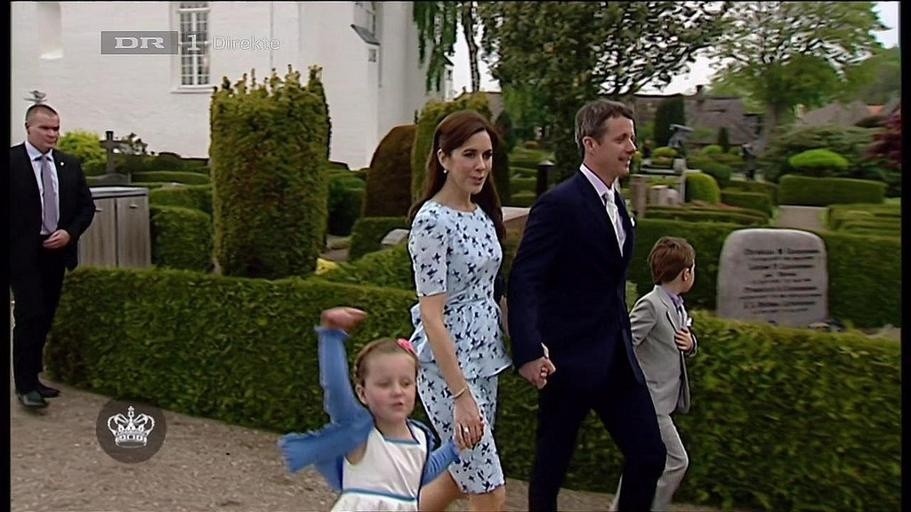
[42,156,58,232]
[603,190,622,252]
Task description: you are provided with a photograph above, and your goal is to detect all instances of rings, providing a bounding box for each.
[463,426,469,434]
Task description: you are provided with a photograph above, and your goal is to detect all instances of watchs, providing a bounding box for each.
[284,306,482,512]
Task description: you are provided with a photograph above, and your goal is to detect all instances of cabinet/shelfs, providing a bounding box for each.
[76,185,151,270]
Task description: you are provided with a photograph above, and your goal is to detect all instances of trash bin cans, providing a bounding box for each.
[77,187,152,267]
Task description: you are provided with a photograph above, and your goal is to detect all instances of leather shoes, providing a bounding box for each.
[17,383,60,408]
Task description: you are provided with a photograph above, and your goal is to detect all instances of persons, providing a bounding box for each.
[9,104,95,409]
[611,236,697,511]
[408,109,549,511]
[507,100,667,512]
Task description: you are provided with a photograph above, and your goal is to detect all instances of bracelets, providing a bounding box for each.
[449,384,470,401]
